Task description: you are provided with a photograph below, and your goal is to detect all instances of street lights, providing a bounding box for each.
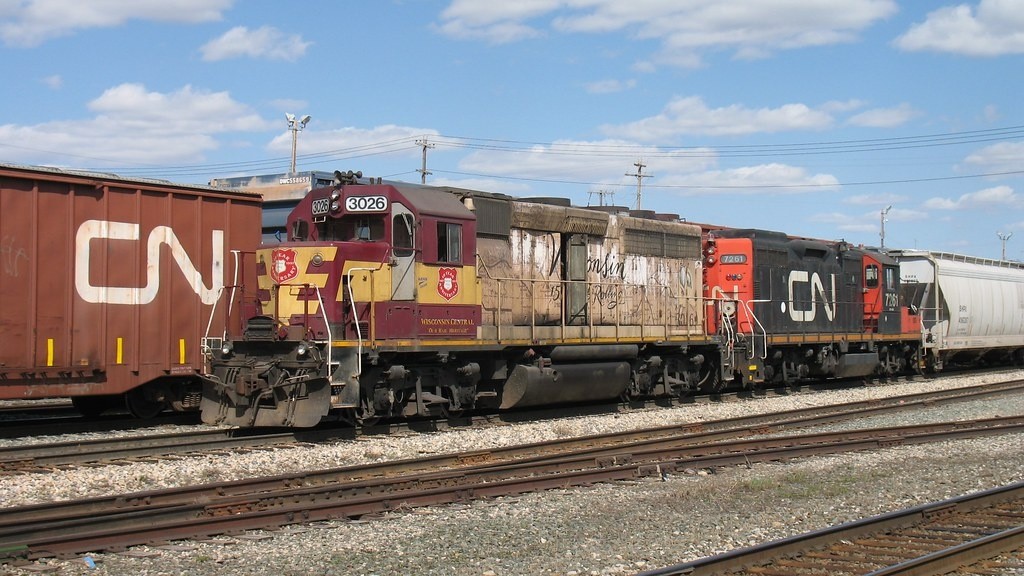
[881,205,892,249]
[997,232,1013,260]
[284,113,312,173]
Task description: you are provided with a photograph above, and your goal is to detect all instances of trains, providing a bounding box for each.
[0,164,303,417]
[190,172,1023,429]
